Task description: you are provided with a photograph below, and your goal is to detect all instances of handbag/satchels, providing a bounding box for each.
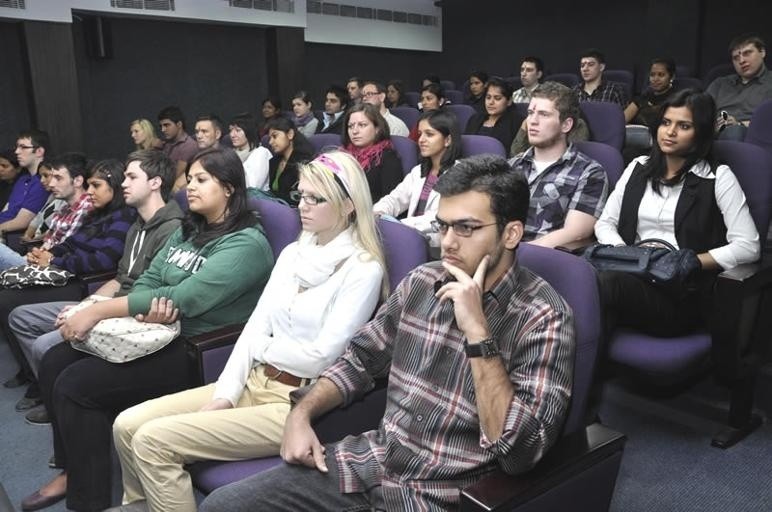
[0,255,76,290]
[580,239,706,296]
[59,294,183,365]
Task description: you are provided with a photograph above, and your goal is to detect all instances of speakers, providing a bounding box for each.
[89,15,112,58]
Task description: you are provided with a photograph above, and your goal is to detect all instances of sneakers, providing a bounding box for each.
[24,408,51,426]
[48,456,57,469]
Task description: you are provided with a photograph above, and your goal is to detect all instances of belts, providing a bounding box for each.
[263,363,310,389]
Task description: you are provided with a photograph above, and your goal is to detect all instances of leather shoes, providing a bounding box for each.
[15,390,44,412]
[4,370,29,388]
[21,466,67,510]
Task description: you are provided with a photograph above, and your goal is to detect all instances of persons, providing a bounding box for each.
[0,28,772,512]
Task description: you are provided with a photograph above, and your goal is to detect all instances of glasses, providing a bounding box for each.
[431,220,498,237]
[290,191,326,206]
[717,110,728,133]
